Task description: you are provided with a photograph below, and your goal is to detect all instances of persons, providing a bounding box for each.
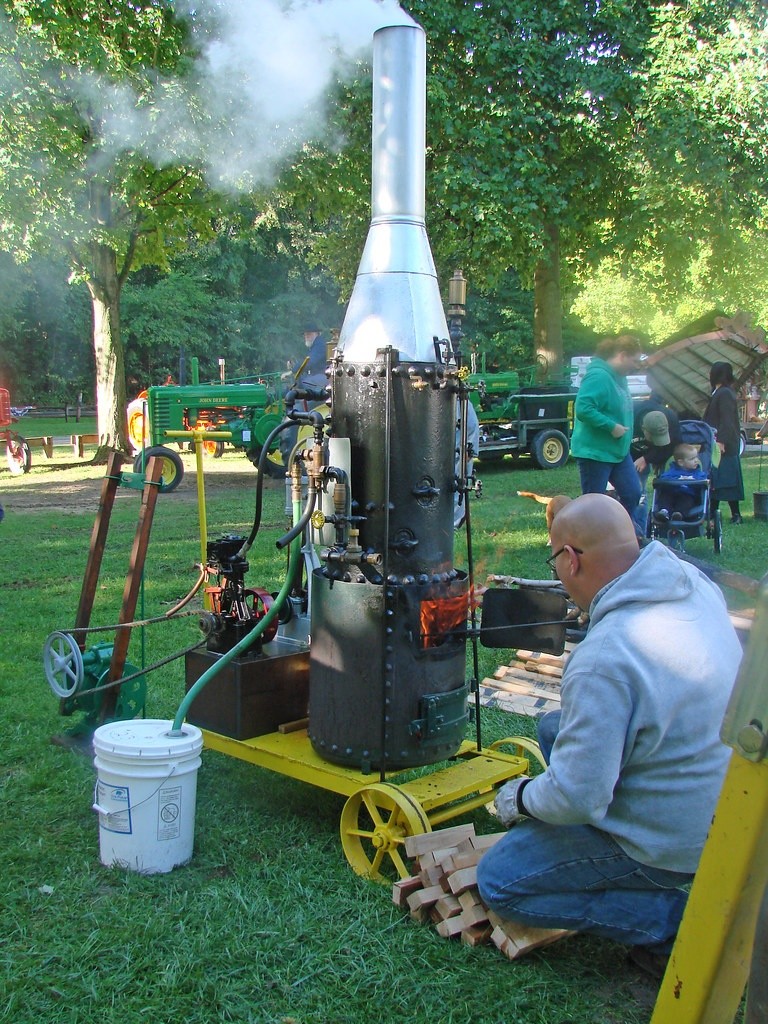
[704,361,746,525]
[566,334,706,539]
[477,495,743,967]
[281,324,327,391]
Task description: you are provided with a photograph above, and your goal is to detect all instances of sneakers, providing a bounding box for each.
[657,509,682,521]
[631,945,666,979]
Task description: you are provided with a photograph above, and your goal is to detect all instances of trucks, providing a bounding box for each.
[570,355,652,400]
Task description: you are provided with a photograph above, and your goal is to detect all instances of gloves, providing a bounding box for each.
[493,774,534,829]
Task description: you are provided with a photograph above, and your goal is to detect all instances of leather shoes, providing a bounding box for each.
[730,513,743,525]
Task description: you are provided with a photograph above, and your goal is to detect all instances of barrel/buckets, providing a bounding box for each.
[92,718,204,874]
[752,491,768,521]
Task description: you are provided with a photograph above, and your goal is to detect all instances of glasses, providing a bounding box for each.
[545,546,585,570]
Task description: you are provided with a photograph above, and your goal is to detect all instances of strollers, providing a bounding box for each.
[647,418,724,556]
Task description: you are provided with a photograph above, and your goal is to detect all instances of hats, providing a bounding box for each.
[642,410,670,447]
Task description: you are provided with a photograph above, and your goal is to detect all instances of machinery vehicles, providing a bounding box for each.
[464,353,580,463]
[131,342,330,493]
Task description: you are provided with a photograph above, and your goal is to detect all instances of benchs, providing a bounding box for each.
[71,434,99,458]
[0,436,54,458]
